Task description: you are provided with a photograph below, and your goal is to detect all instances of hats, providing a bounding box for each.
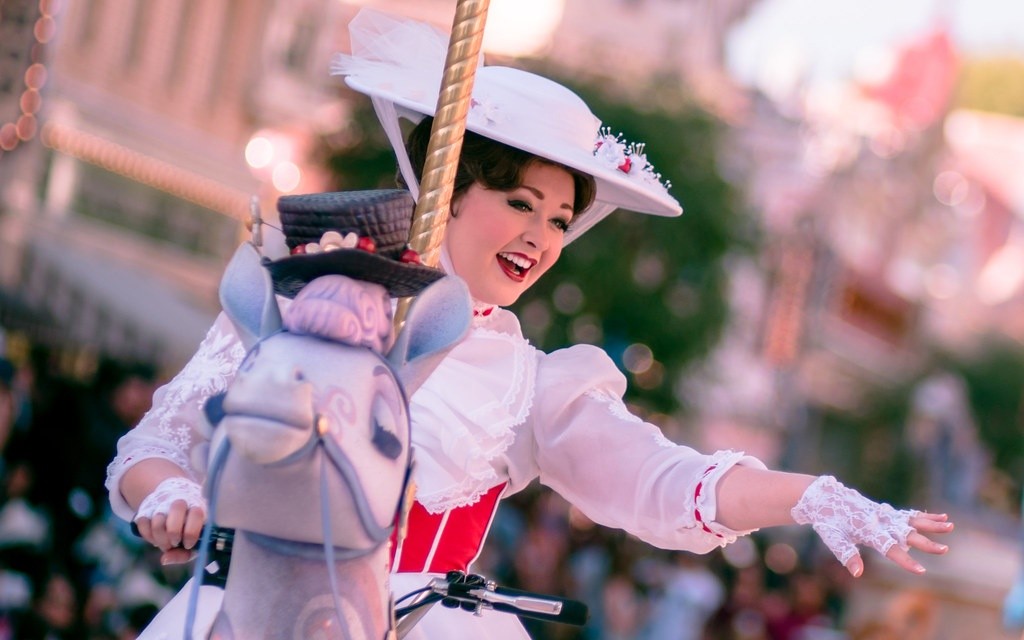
[328,3,684,218]
[258,185,447,302]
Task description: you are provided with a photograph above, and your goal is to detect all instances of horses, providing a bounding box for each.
[199,236,414,640]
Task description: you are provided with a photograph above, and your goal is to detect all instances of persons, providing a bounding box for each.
[1,302,848,640]
[104,5,955,640]
